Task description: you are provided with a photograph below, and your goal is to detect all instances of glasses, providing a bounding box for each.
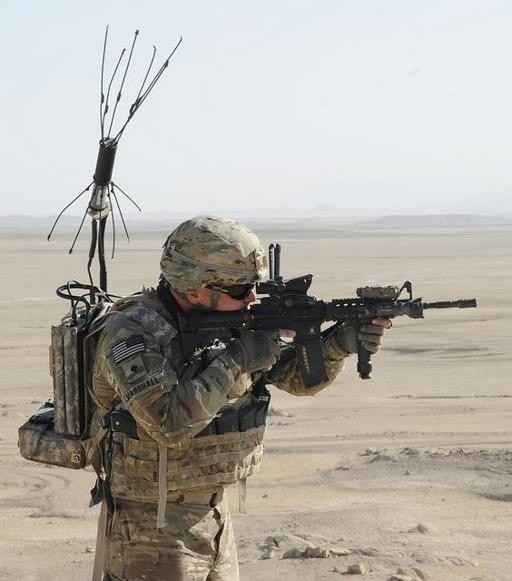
[205,283,255,300]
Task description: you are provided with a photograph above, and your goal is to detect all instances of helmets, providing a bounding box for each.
[159,214,270,296]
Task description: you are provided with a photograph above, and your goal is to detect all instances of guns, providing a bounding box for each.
[178,243,477,389]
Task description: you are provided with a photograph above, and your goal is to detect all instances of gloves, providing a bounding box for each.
[336,318,394,355]
[225,328,283,373]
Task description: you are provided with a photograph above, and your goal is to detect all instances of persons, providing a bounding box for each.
[17,213,392,581]
